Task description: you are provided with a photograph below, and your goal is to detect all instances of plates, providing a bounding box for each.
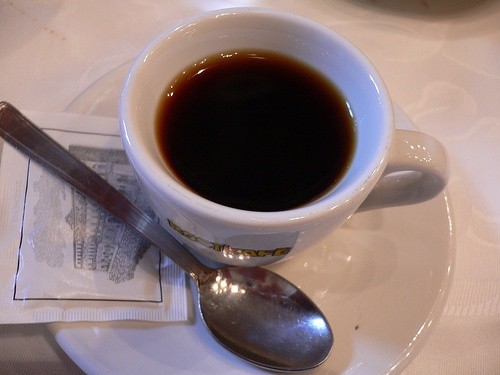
[25,49,459,374]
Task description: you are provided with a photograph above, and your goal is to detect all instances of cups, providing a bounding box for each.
[118,6,448,272]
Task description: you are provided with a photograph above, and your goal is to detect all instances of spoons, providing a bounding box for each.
[0,102,335,371]
